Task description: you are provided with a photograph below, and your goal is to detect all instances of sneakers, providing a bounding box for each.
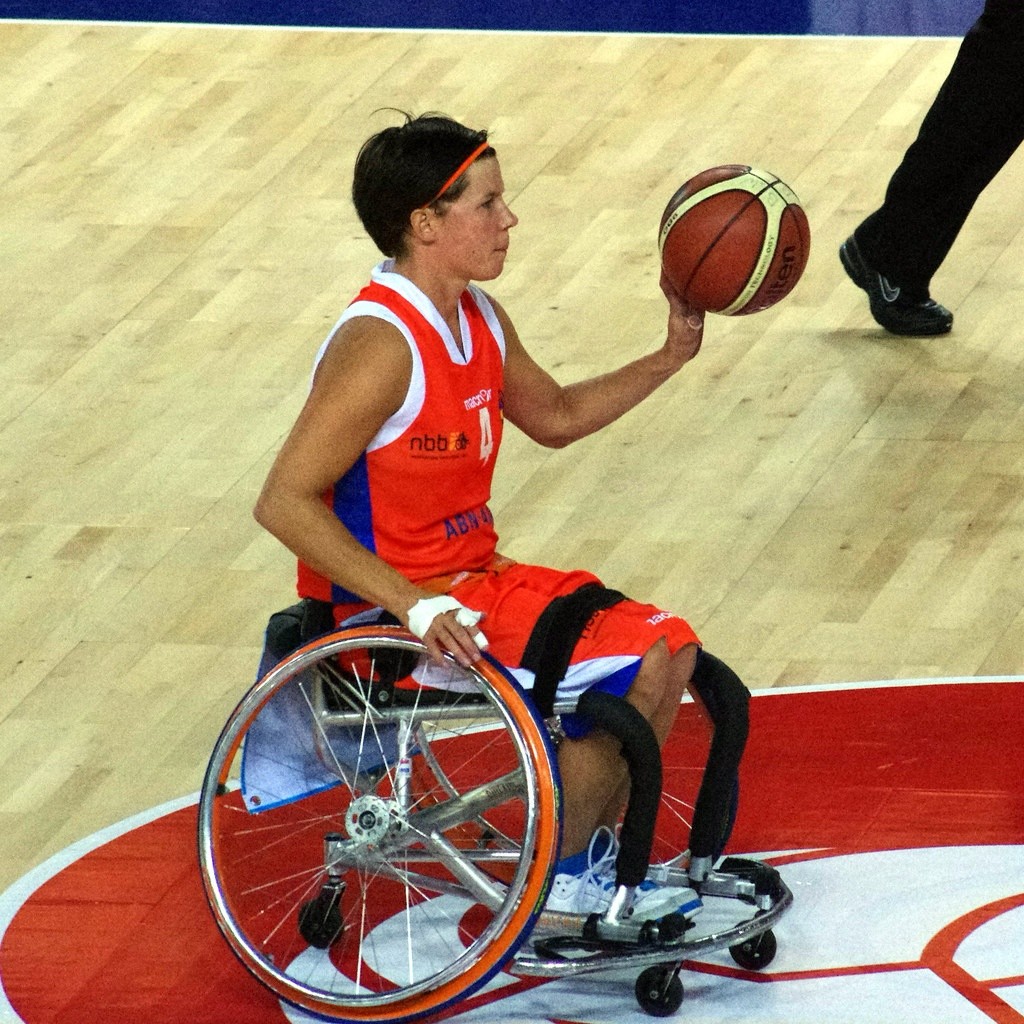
[542,823,704,926]
[838,228,955,336]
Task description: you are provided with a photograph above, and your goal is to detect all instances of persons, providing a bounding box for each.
[841,0,1024,336]
[252,105,704,925]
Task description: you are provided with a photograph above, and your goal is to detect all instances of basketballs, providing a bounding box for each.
[657,162,814,320]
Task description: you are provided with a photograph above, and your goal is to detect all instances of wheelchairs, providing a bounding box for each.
[194,597,797,1023]
